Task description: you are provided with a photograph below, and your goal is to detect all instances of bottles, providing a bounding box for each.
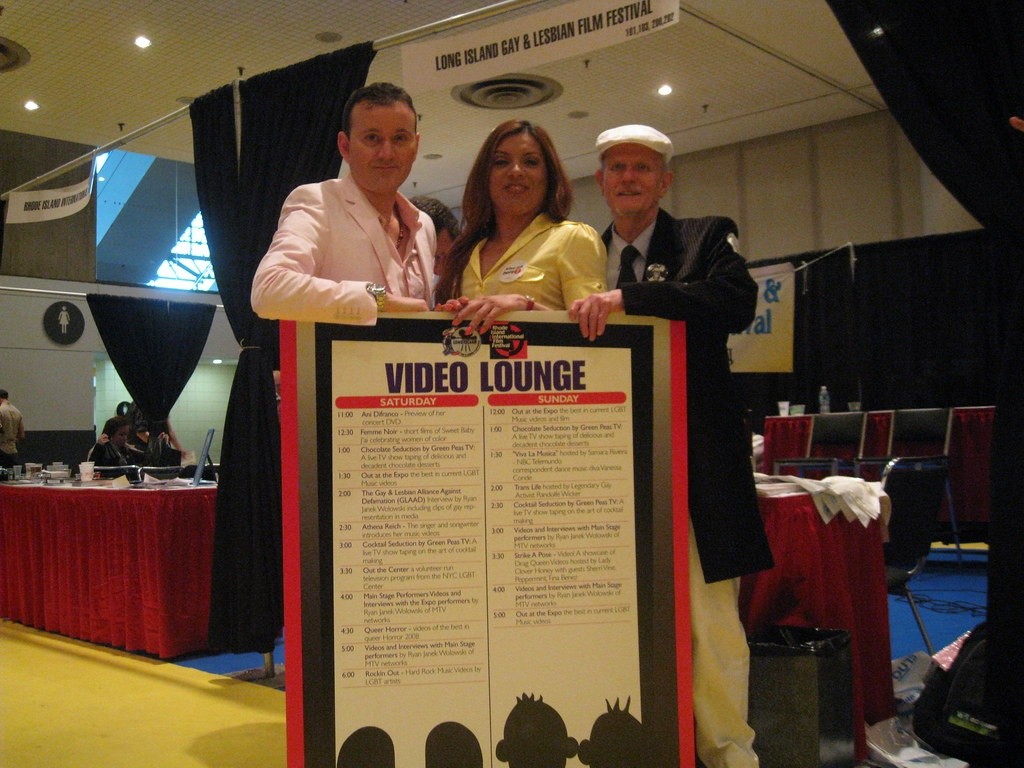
[820,386,829,413]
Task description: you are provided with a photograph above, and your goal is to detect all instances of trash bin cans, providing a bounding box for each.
[747,624,856,768]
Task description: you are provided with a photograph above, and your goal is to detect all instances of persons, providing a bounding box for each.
[89,400,190,479]
[0,389,24,468]
[434,117,606,337]
[568,123,775,768]
[251,81,459,316]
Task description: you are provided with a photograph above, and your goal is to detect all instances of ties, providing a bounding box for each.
[615,245,641,289]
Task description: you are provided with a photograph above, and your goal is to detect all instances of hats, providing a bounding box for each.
[595,124,674,164]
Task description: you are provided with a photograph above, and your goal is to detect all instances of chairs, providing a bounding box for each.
[874,451,954,658]
[770,407,865,478]
[855,408,965,554]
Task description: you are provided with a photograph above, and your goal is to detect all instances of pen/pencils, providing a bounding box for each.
[189,482,214,485]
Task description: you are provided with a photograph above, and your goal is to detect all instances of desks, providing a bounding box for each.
[734,479,897,725]
[0,477,225,658]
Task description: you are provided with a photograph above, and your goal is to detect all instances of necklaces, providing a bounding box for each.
[395,204,404,250]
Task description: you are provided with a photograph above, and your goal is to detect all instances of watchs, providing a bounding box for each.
[526,295,534,310]
[367,282,385,311]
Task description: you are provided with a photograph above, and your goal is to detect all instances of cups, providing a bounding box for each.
[778,401,789,416]
[7,462,42,484]
[79,462,95,482]
[848,402,860,412]
